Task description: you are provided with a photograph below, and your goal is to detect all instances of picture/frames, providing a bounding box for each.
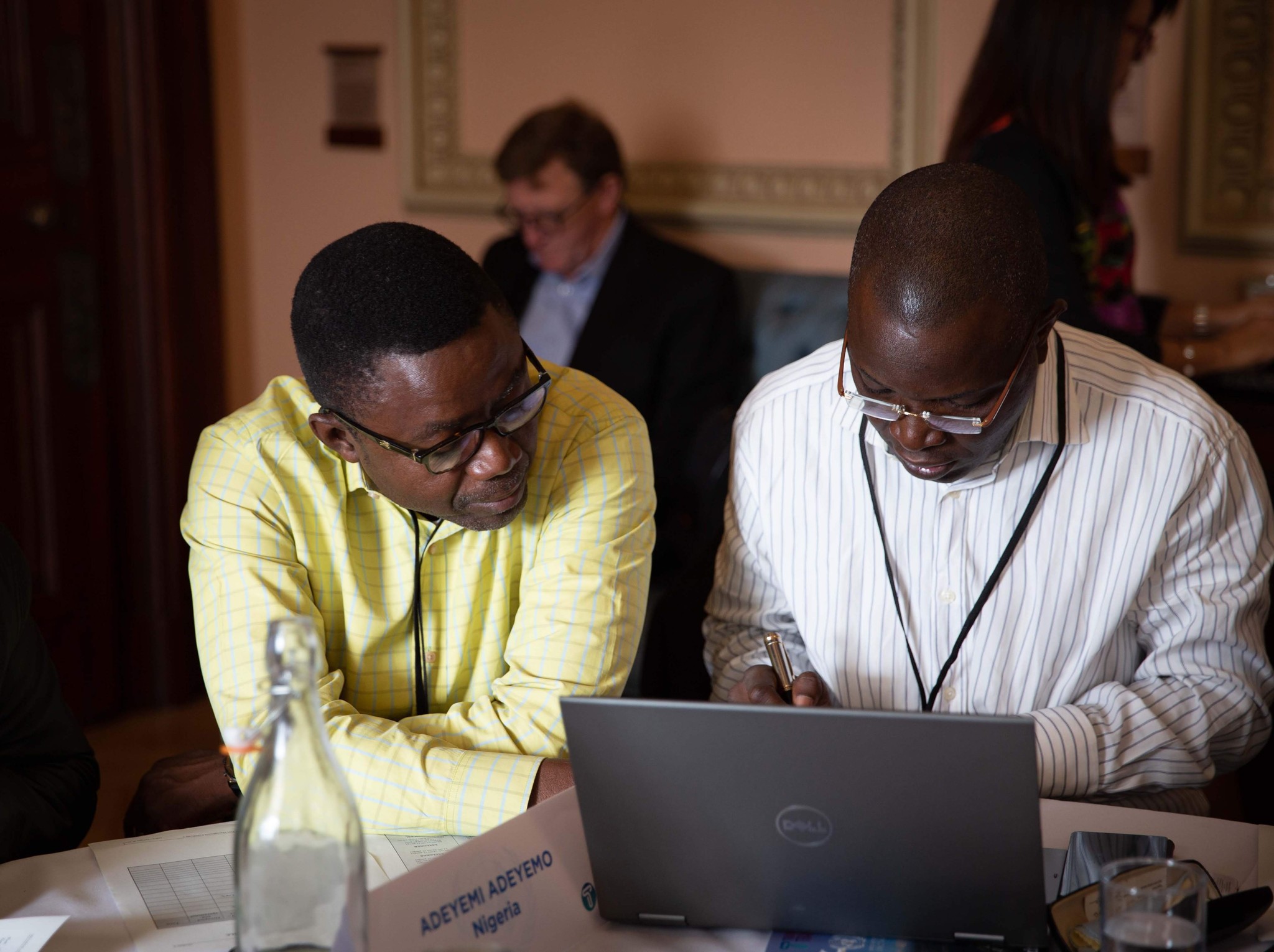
[1169,0,1274,260]
[389,1,936,238]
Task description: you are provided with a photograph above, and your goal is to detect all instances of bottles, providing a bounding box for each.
[231,615,369,952]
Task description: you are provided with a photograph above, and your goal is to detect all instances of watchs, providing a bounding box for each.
[218,740,241,797]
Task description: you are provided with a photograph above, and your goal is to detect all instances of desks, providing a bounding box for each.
[0,793,1274,951]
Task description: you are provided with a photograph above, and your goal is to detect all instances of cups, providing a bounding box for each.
[1098,857,1209,952]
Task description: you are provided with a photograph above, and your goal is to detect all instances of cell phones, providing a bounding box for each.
[1057,831,1168,897]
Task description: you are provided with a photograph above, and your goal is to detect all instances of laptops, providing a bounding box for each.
[559,696,1068,952]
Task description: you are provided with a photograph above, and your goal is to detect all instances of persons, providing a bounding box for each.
[701,162,1274,805]
[121,221,656,852]
[0,523,103,868]
[938,0,1274,368]
[473,100,730,706]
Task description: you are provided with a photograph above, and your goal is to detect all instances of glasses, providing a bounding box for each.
[495,189,587,232]
[317,336,553,476]
[837,327,1033,434]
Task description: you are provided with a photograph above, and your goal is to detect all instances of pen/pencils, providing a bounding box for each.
[763,631,794,704]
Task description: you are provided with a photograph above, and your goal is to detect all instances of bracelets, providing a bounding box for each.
[1182,338,1196,377]
[1192,300,1209,336]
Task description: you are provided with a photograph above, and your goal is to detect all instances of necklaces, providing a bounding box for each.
[404,503,448,718]
[859,327,1068,712]
